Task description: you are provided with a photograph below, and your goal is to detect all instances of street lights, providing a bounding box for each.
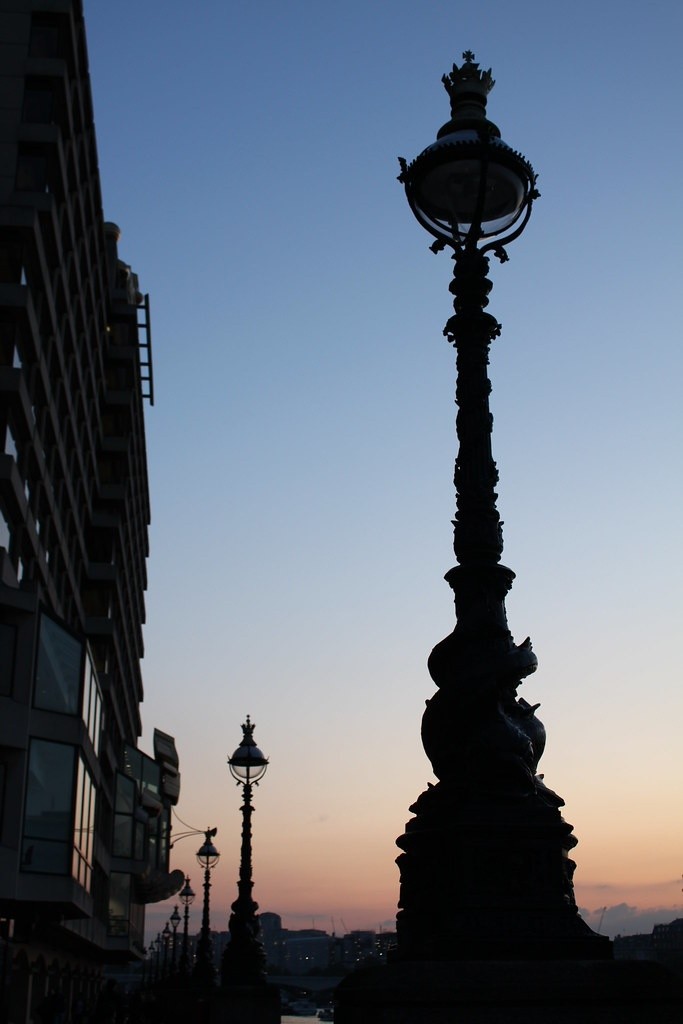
[160,921,173,980]
[201,714,283,1024]
[176,874,198,984]
[140,947,147,984]
[169,903,183,977]
[154,933,163,983]
[389,48,618,1024]
[148,940,156,982]
[194,827,220,988]
